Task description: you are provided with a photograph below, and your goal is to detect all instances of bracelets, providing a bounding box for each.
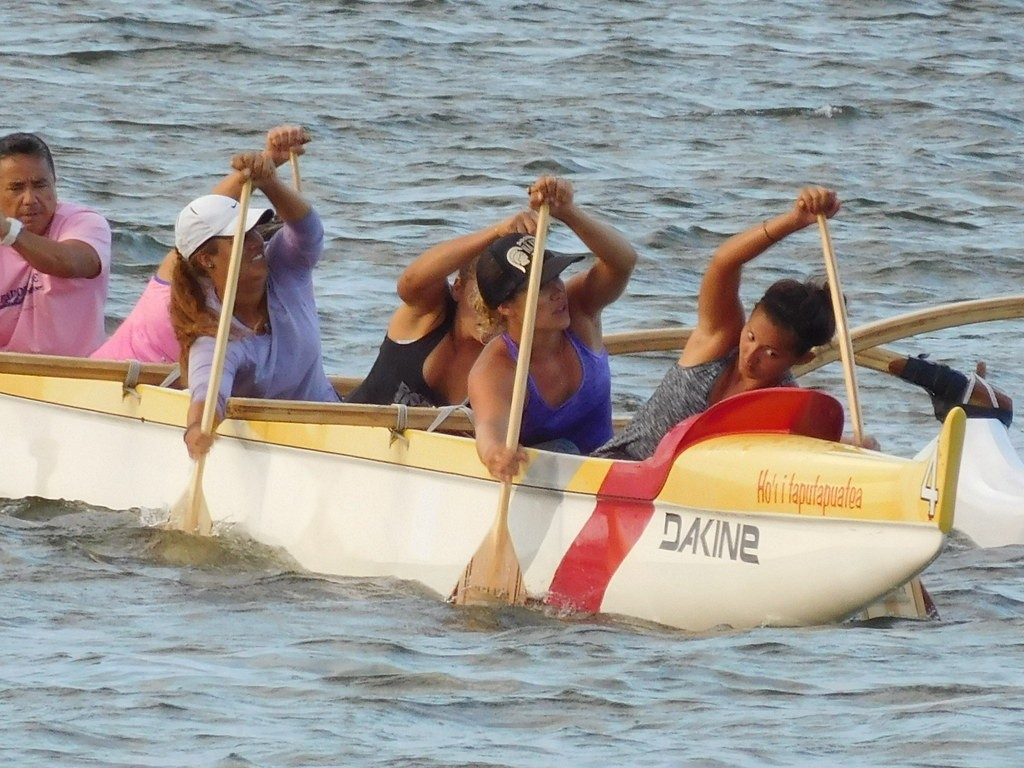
[763,220,778,242]
[0,217,24,246]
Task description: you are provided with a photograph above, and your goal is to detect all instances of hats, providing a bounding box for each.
[476,233,585,309]
[174,195,274,261]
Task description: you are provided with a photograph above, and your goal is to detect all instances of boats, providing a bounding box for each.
[0,351,967,632]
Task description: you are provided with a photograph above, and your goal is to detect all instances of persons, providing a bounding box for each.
[583,185,881,462]
[466,174,638,484]
[345,211,539,409]
[87,124,313,364]
[168,150,343,462]
[0,131,113,359]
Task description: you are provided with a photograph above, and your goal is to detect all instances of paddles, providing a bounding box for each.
[800,188,942,623]
[444,183,562,610]
[164,158,260,540]
